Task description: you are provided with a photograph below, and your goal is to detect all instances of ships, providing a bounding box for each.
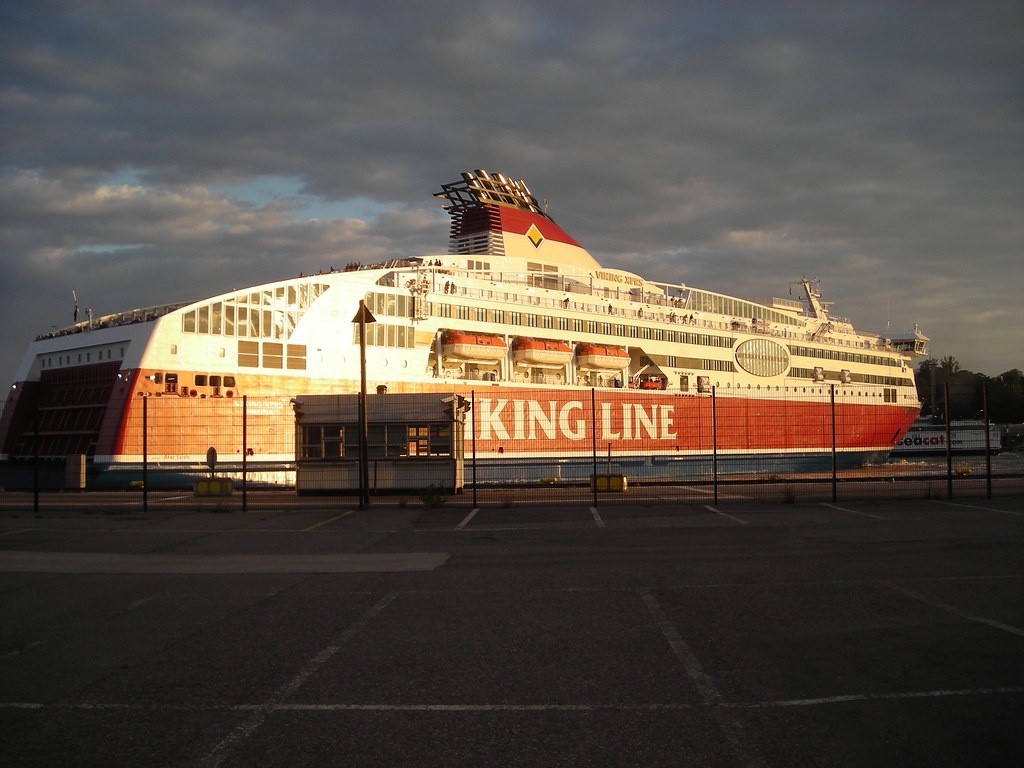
[0,169,932,489]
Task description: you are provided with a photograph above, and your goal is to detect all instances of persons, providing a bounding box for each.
[299,258,455,295]
[432,283,759,389]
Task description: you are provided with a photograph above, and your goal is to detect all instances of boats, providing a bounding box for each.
[892,397,1003,455]
[443,333,508,362]
[513,336,576,367]
[577,344,632,371]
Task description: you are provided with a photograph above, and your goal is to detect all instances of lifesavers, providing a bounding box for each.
[411,279,416,285]
[405,281,411,288]
[417,288,422,295]
